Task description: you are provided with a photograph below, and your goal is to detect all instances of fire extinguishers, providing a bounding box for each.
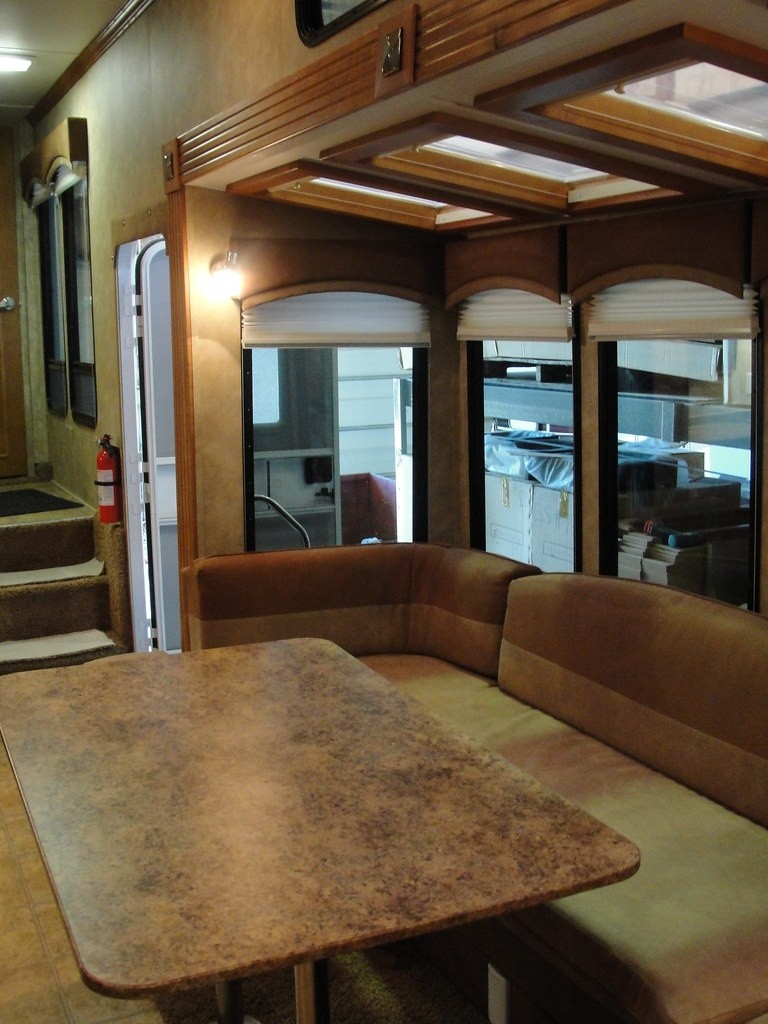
[93,434,123,525]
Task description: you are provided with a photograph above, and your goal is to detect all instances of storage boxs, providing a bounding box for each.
[661,449,705,482]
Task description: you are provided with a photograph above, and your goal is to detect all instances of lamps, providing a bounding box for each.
[210,251,237,277]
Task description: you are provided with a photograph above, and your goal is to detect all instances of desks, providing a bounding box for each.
[0,638,640,1024]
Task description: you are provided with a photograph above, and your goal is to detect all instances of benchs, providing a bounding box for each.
[179,544,768,1024]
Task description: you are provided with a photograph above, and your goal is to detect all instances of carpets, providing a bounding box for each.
[0,488,85,518]
[0,629,116,664]
[0,557,105,587]
